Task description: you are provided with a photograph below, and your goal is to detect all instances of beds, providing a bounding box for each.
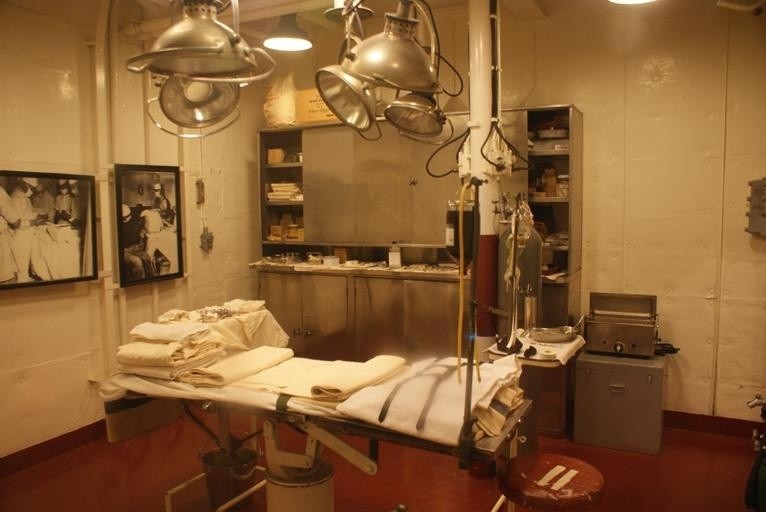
[107,353,534,511]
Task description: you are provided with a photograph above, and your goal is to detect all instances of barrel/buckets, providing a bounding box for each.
[199,448,257,510]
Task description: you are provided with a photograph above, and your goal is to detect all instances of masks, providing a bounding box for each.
[153,191,163,199]
[123,216,132,223]
[24,185,33,198]
[60,188,69,195]
[71,187,81,196]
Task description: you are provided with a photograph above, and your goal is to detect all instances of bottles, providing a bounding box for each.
[541,168,556,197]
[388,240,402,269]
[443,197,475,276]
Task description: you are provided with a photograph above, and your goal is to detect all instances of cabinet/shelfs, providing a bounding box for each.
[240,103,584,355]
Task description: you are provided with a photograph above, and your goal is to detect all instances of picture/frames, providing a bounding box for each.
[1,163,186,290]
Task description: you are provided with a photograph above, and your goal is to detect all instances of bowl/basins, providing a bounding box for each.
[529,325,579,344]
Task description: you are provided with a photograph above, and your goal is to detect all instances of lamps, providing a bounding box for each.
[123,0,276,140]
[262,13,313,52]
[313,1,464,143]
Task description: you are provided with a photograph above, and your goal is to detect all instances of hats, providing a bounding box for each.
[58,179,66,186]
[35,184,44,191]
[153,183,161,189]
[122,203,131,217]
[22,177,38,188]
[68,179,79,184]
